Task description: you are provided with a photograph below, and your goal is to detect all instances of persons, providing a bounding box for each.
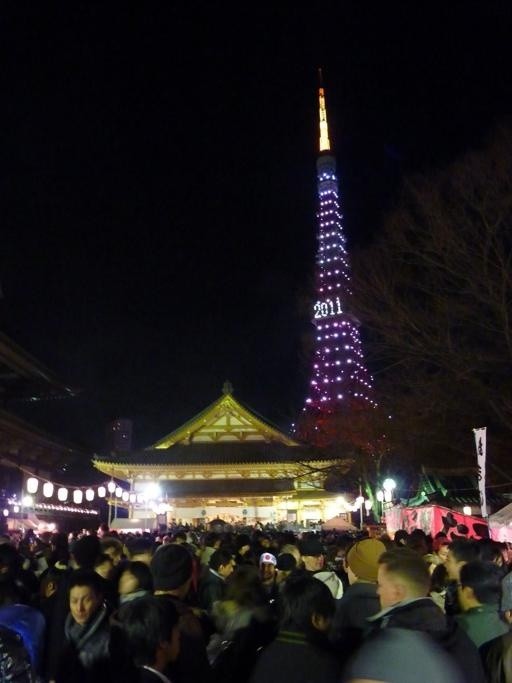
[1,518,512,682]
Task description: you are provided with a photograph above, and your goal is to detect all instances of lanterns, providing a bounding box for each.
[327,479,396,514]
[26,477,143,504]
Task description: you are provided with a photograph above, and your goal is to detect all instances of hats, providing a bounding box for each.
[351,627,461,683]
[500,571,512,611]
[347,539,387,581]
[313,572,343,600]
[151,544,193,592]
[49,533,68,548]
[236,534,250,546]
[299,540,326,555]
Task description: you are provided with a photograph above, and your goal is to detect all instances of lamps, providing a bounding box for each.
[27,477,136,504]
[463,506,472,515]
